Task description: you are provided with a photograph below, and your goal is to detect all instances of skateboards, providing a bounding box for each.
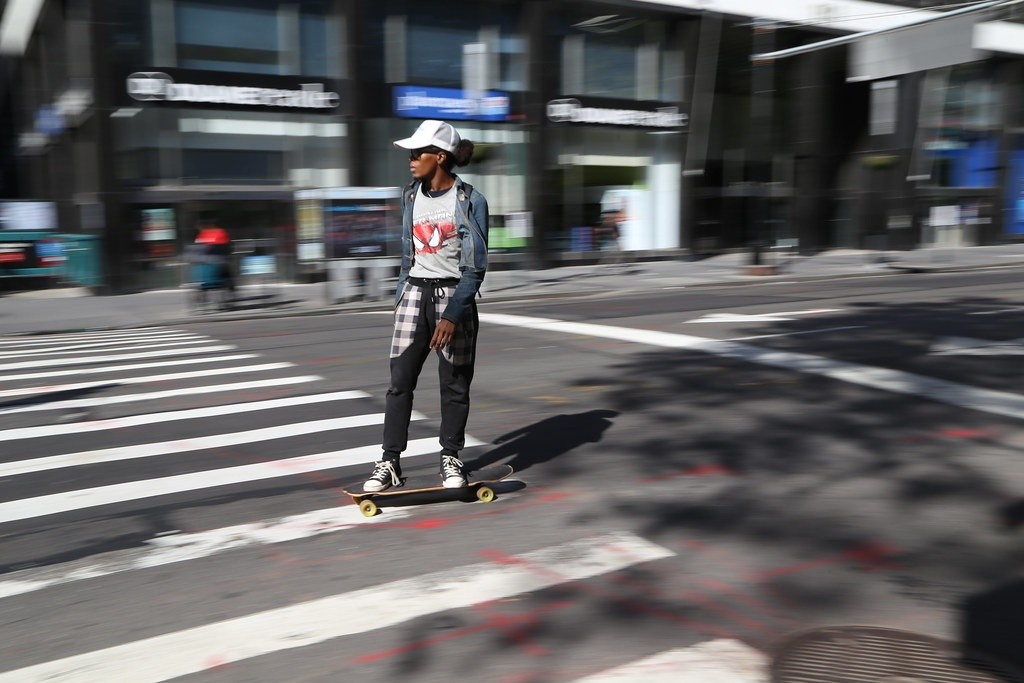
[343,465,513,517]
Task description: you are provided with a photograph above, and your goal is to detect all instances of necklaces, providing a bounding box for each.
[424,186,433,198]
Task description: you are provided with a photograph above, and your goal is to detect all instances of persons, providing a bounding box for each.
[362,121,489,490]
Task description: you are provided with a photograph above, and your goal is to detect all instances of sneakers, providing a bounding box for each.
[362,457,402,491]
[439,453,466,489]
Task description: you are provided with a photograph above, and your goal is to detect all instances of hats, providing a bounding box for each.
[393,120,461,156]
